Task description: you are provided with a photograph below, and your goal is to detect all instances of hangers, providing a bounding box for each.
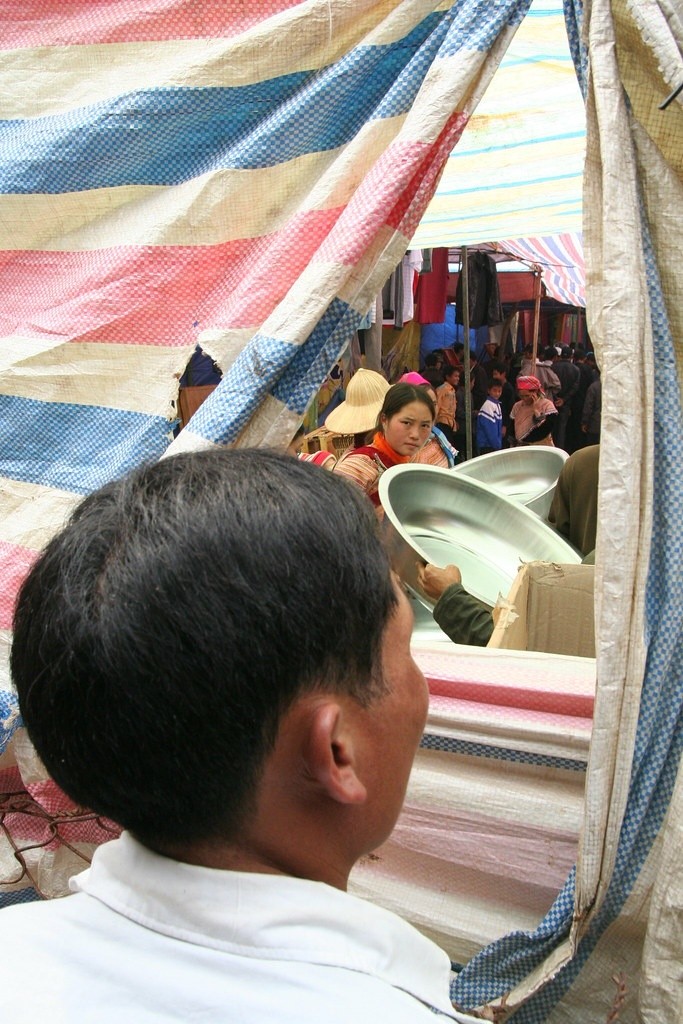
[476,247,484,255]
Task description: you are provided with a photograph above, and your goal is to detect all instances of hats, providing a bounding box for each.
[296,451,336,468]
[398,371,431,387]
[484,329,498,344]
[325,367,398,435]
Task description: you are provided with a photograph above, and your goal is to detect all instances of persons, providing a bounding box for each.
[506,375,560,448]
[284,365,461,524]
[0,447,494,1024]
[414,548,595,647]
[547,410,602,557]
[476,377,504,456]
[419,333,602,442]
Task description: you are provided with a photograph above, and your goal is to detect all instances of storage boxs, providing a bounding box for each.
[180,385,216,427]
[485,558,597,660]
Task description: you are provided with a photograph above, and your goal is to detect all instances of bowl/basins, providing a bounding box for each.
[450,445,572,520]
[378,463,583,615]
